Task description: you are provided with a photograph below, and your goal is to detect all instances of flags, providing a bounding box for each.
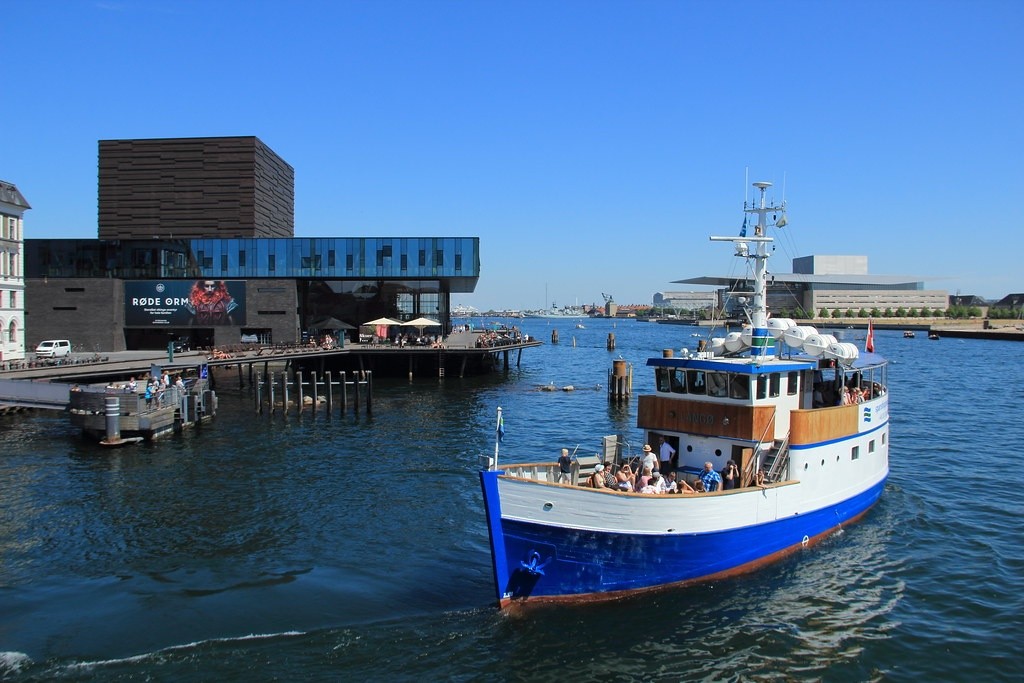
[739,216,748,237]
[862,316,875,352]
[496,412,505,443]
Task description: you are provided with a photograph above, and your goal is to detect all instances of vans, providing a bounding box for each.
[34,339,72,360]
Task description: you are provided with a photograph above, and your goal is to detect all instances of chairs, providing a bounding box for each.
[197,337,321,360]
[361,339,398,349]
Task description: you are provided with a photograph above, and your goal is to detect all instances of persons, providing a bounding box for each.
[635,445,660,485]
[212,347,232,358]
[390,331,445,348]
[658,436,675,475]
[697,461,724,492]
[838,371,881,405]
[747,468,769,490]
[71,370,186,410]
[814,383,825,407]
[477,324,528,348]
[635,466,694,495]
[368,332,379,348]
[320,334,340,350]
[452,322,470,333]
[721,459,740,490]
[558,447,576,487]
[190,280,238,325]
[586,461,635,492]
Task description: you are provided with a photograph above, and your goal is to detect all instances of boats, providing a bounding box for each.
[904,330,915,338]
[474,166,892,616]
[929,334,941,340]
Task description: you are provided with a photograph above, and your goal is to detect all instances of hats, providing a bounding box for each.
[652,472,662,478]
[643,445,651,452]
[604,462,613,467]
[595,464,605,472]
[727,460,736,467]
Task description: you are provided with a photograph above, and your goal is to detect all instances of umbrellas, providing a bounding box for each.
[309,315,357,332]
[408,315,442,336]
[363,317,402,337]
